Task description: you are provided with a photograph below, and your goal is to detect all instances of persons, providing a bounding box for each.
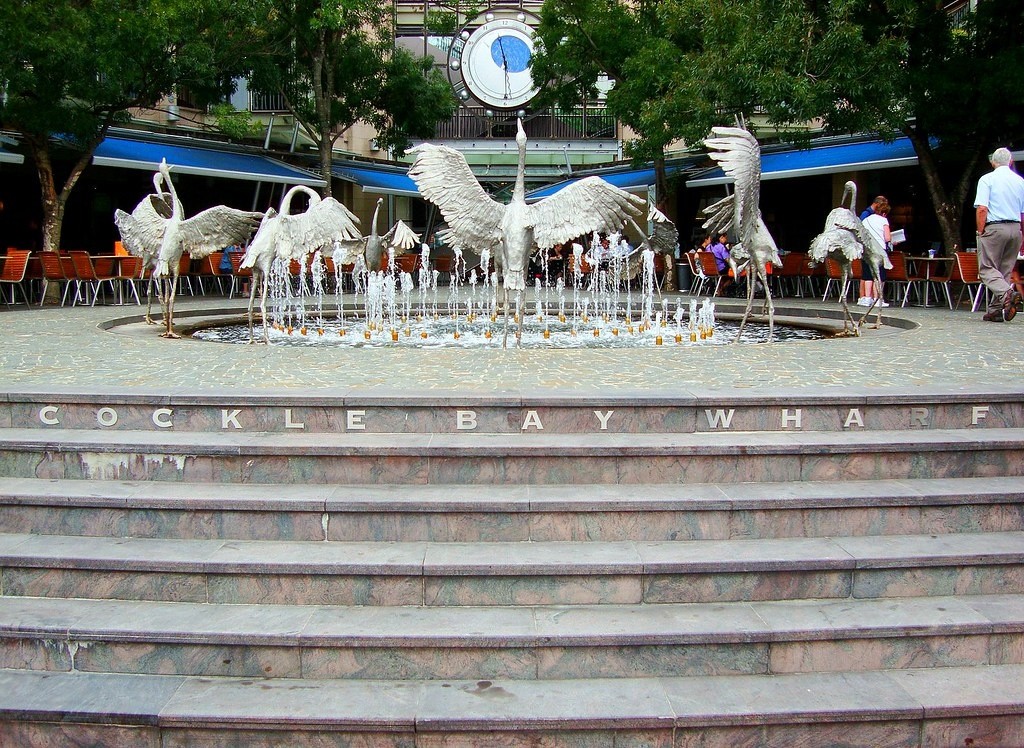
[973,148,1023,322]
[694,235,713,258]
[856,196,900,307]
[861,202,891,308]
[547,244,563,285]
[596,239,612,262]
[614,236,634,255]
[218,243,250,298]
[705,232,732,297]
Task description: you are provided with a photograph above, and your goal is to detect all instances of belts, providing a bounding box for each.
[985,220,1021,227]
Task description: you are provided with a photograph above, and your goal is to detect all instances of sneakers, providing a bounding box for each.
[857,297,873,307]
[871,298,890,308]
[1004,289,1022,321]
[983,310,1004,322]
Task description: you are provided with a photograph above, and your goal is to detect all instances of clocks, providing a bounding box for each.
[460,18,550,111]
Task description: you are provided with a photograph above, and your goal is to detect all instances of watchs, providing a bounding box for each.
[976,231,981,236]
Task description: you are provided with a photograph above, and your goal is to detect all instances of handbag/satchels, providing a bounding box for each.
[885,245,892,255]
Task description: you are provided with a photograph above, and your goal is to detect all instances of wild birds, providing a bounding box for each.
[808,179,895,337]
[317,197,424,277]
[115,156,266,341]
[701,111,783,346]
[403,117,680,351]
[238,184,365,346]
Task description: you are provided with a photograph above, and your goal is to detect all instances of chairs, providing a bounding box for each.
[1,209,985,318]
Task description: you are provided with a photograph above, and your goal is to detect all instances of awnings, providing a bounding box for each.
[1,126,328,190]
[523,162,694,203]
[686,131,1023,192]
[312,161,496,201]
